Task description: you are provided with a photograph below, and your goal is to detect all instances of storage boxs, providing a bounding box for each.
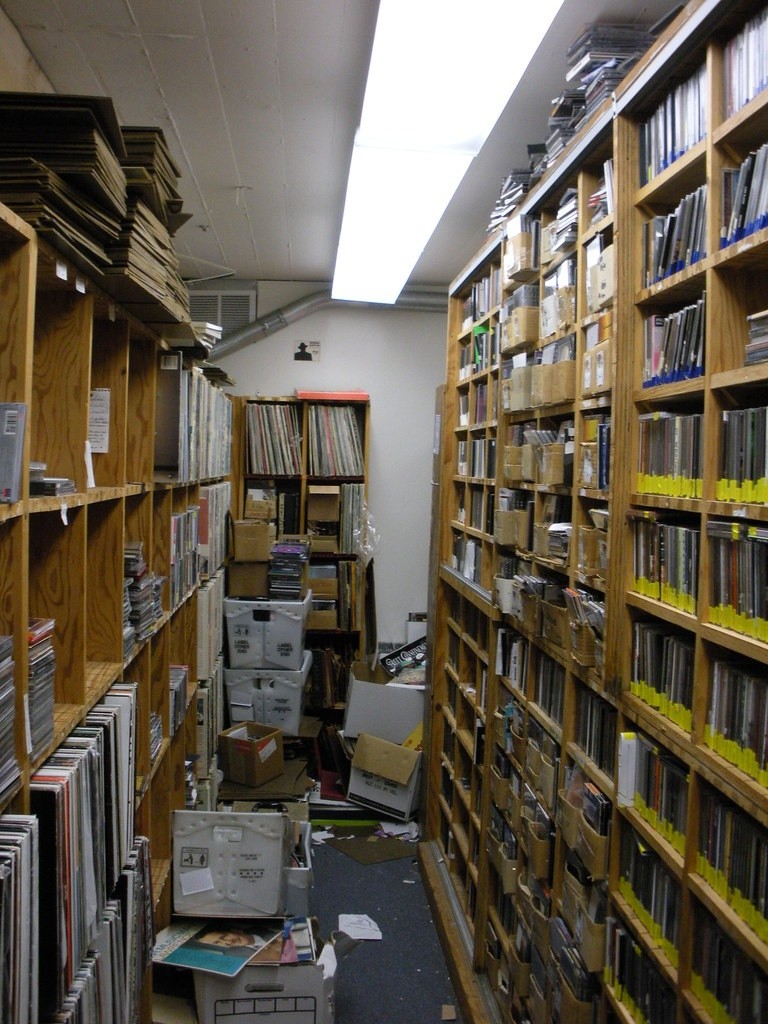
[343,661,426,744]
[228,557,269,597]
[218,721,284,788]
[190,917,361,1024]
[307,485,340,522]
[173,809,294,919]
[223,650,314,737]
[223,588,312,671]
[306,600,337,630]
[232,789,310,824]
[233,518,270,562]
[283,821,314,918]
[344,733,422,823]
[305,565,338,599]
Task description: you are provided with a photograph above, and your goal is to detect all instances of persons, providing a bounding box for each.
[196,924,285,954]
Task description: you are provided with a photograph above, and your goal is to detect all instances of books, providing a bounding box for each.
[268,538,307,604]
[246,401,364,475]
[337,560,363,631]
[0,85,281,1023]
[414,5,766,1024]
[287,637,359,790]
[340,481,366,559]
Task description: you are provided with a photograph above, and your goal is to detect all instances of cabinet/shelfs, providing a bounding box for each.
[416,0,768,1024]
[231,389,373,661]
[0,201,231,1024]
